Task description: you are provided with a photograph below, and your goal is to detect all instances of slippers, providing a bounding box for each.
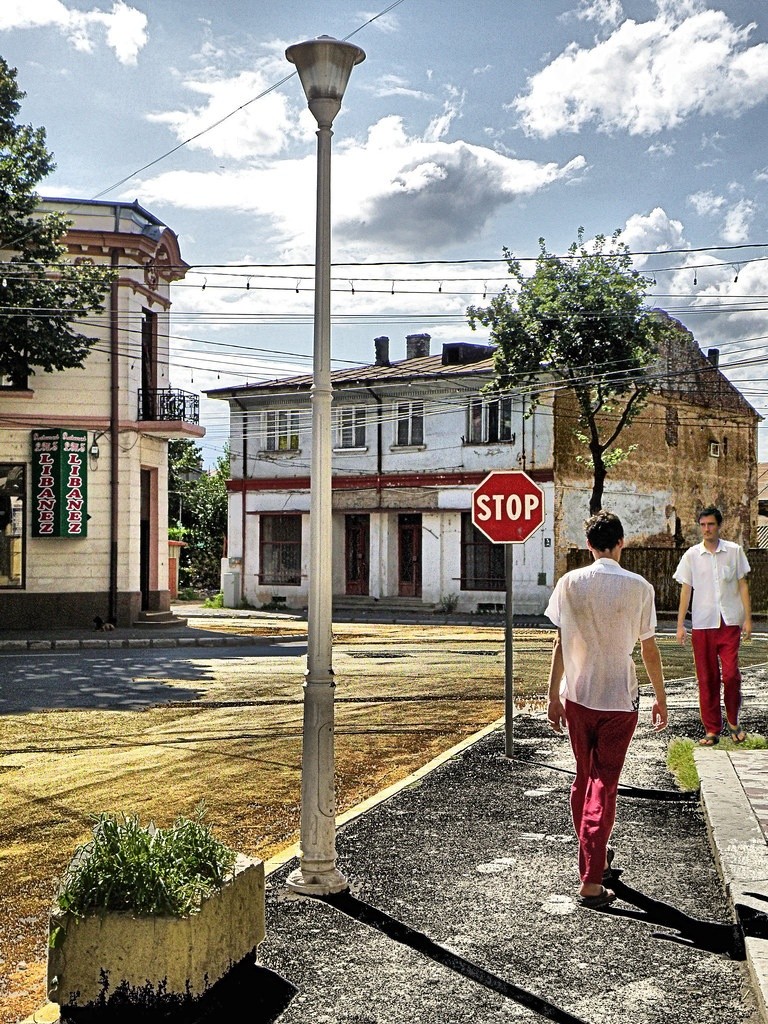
[726,722,745,743]
[583,886,614,907]
[602,845,614,876]
[699,735,720,746]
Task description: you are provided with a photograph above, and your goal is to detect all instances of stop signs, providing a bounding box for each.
[471,469,546,549]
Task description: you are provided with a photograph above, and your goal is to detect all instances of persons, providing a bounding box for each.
[544,510,667,897]
[673,508,752,746]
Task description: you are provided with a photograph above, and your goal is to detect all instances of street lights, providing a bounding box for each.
[281,25,368,906]
